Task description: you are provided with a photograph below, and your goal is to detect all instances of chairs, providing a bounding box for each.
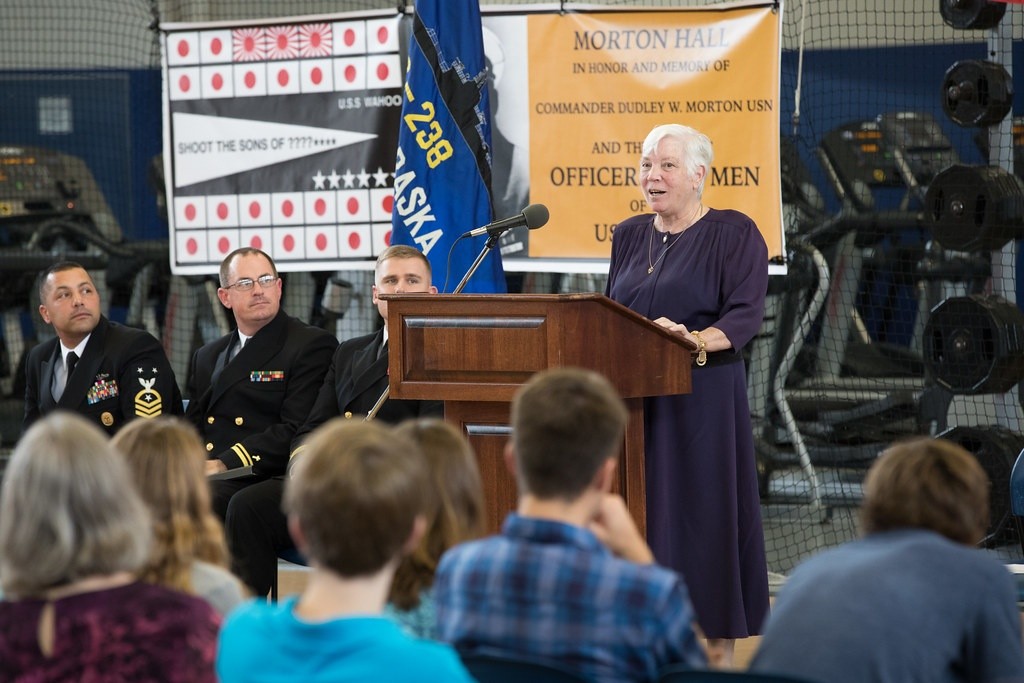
[659,668,804,683]
[459,649,587,683]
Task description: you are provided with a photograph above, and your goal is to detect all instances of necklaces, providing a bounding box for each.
[648,206,700,274]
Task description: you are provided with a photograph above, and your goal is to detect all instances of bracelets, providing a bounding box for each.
[691,331,707,366]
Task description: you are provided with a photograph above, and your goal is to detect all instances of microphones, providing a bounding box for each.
[461,204,549,238]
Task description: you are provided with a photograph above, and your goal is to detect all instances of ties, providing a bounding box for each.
[378,339,389,360]
[65,350,80,390]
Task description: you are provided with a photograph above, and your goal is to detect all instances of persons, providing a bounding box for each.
[225,245,443,608]
[383,420,482,640]
[110,418,244,620]
[436,369,709,683]
[0,413,224,683]
[604,124,772,669]
[748,439,1024,683]
[22,261,185,438]
[185,248,340,524]
[215,420,475,683]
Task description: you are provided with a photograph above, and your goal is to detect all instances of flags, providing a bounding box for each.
[392,0,508,295]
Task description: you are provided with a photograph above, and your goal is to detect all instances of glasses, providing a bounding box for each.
[225,275,278,294]
